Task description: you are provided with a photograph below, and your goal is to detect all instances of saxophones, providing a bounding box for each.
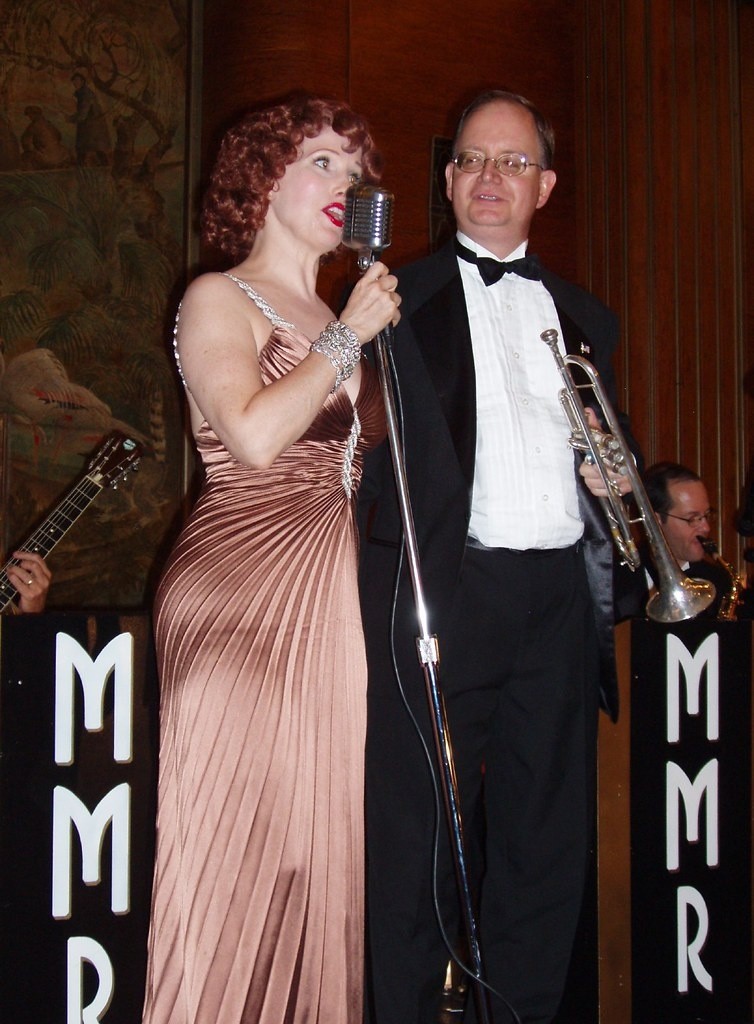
[696,535,744,622]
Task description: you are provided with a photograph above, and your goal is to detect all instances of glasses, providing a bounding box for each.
[450,150,545,177]
[657,507,718,529]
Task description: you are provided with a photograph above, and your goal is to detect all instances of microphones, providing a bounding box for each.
[340,182,394,340]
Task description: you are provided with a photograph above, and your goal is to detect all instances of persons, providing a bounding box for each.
[614,460,732,623]
[141,92,403,1024]
[357,89,643,1024]
[0,550,52,614]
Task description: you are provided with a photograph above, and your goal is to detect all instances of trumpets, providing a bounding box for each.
[543,329,717,623]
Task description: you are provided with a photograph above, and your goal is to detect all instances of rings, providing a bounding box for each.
[27,578,34,585]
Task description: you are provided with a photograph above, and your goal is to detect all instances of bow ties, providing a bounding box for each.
[452,234,546,287]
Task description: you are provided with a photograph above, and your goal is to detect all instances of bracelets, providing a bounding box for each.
[309,320,361,392]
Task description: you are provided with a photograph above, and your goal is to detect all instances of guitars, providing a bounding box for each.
[0,428,142,613]
